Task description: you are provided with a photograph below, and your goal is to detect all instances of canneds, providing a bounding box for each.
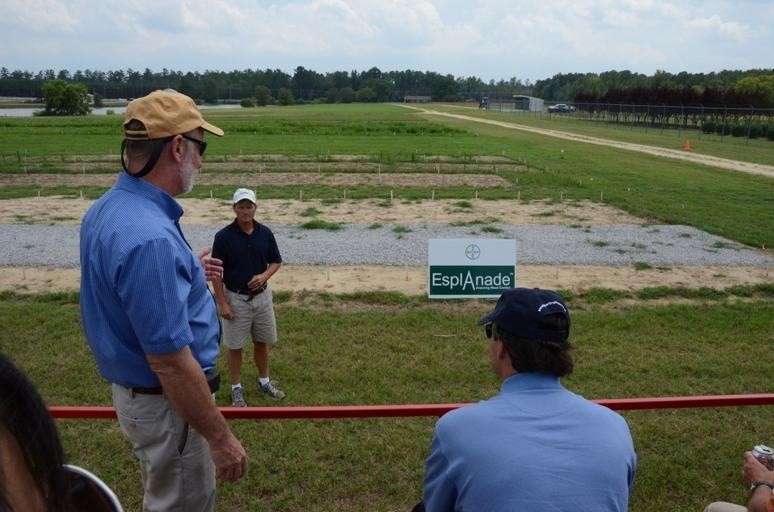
[750,444,774,470]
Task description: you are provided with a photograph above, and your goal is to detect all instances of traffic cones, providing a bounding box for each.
[684,139,690,150]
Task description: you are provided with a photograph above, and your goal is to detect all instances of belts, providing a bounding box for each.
[225,283,267,295]
[113,374,221,394]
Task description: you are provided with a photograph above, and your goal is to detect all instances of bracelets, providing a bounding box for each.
[746,479,773,497]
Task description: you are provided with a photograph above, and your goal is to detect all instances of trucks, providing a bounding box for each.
[79,93,96,108]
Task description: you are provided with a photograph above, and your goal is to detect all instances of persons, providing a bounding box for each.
[407,287,640,512]
[704,447,774,512]
[1,351,67,512]
[211,187,288,406]
[80,90,250,512]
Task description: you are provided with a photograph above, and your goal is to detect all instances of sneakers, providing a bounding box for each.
[232,387,247,407]
[257,382,285,400]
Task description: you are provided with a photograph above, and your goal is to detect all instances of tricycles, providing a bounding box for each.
[479,97,489,109]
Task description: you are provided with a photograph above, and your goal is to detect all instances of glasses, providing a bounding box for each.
[182,134,207,156]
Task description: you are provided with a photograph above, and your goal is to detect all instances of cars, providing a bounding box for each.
[547,104,576,113]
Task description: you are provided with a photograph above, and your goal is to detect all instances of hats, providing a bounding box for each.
[123,90,224,140]
[232,188,257,206]
[477,288,570,340]
